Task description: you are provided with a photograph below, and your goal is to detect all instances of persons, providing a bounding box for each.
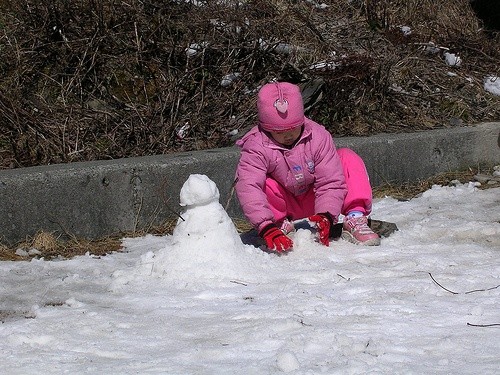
[234,82,380,252]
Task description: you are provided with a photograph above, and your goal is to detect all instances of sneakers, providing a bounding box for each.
[258,217,295,250]
[341,216,381,246]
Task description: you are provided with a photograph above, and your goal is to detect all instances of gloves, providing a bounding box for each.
[258,223,293,251]
[309,214,330,247]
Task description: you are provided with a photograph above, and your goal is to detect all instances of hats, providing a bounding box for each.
[257,78,305,132]
